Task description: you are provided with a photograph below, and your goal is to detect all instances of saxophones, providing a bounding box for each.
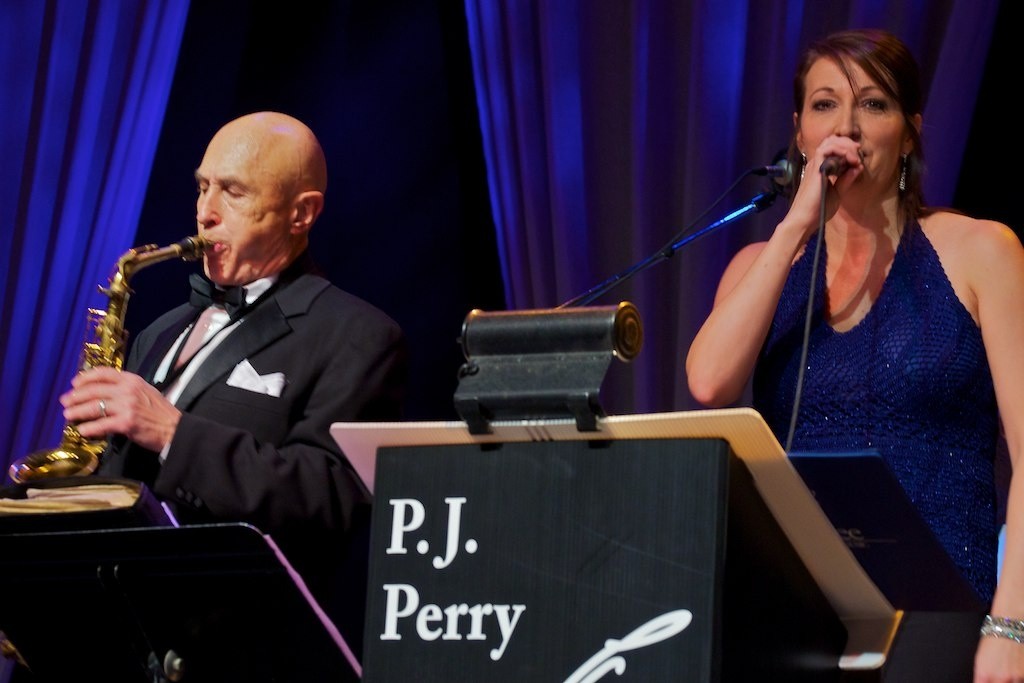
[6,233,217,488]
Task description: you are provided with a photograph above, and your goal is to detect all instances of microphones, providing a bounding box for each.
[819,155,849,176]
[753,159,793,186]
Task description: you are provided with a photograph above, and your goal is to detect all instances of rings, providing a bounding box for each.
[98,399,106,418]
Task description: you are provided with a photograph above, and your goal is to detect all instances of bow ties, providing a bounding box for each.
[189,273,248,321]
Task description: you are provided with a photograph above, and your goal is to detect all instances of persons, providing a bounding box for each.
[60,112,399,630]
[686,31,1023,681]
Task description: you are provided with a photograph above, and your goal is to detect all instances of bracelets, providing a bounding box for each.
[979,614,1024,645]
